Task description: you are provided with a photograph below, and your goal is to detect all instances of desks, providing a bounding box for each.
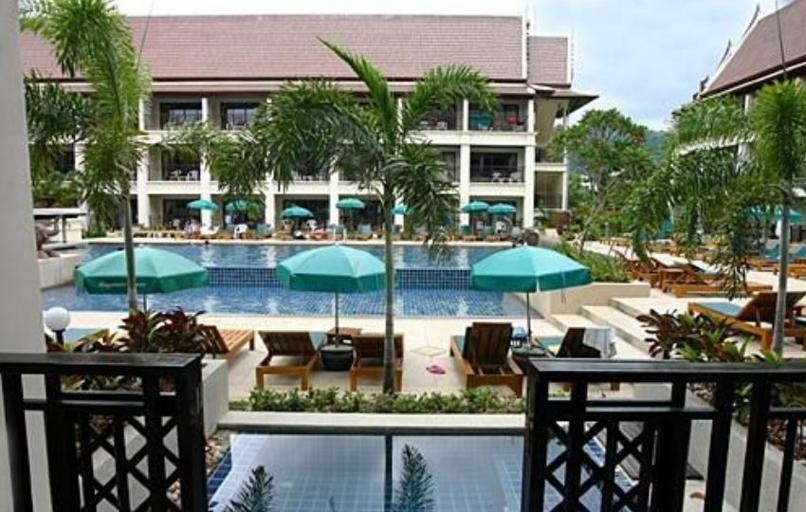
[326,327,362,343]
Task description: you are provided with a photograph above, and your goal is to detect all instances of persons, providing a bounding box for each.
[169,217,200,239]
[282,217,337,240]
[339,215,403,241]
[411,218,463,242]
[225,212,272,239]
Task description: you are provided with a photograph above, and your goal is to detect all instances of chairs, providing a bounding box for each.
[448,320,525,396]
[351,331,403,392]
[601,237,806,353]
[191,220,512,241]
[534,326,617,393]
[195,323,258,361]
[252,330,327,391]
[44,326,110,352]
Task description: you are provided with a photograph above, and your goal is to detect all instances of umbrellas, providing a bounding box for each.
[488,203,516,218]
[391,206,412,214]
[73,242,213,318]
[744,202,803,226]
[461,202,491,215]
[185,199,220,216]
[336,197,367,220]
[279,206,315,221]
[469,237,592,340]
[224,200,264,214]
[276,236,399,347]
